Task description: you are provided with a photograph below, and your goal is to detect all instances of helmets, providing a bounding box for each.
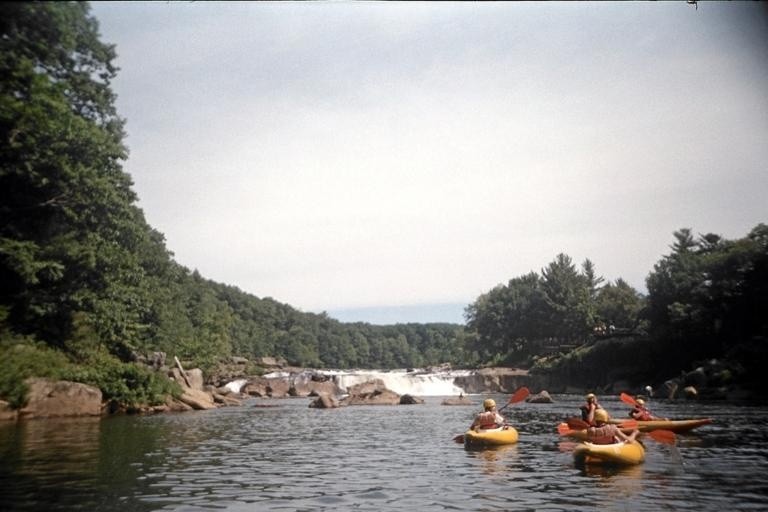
[484,398,496,409]
[636,399,645,407]
[586,393,596,401]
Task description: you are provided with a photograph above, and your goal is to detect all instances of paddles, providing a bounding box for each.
[620,392,663,419]
[453,387,529,440]
[559,417,676,444]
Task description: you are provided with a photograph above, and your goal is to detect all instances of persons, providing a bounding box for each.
[629,399,668,421]
[559,408,640,444]
[469,399,506,430]
[579,393,610,424]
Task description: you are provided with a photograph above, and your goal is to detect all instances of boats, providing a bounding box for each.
[557,418,716,466]
[467,424,519,446]
